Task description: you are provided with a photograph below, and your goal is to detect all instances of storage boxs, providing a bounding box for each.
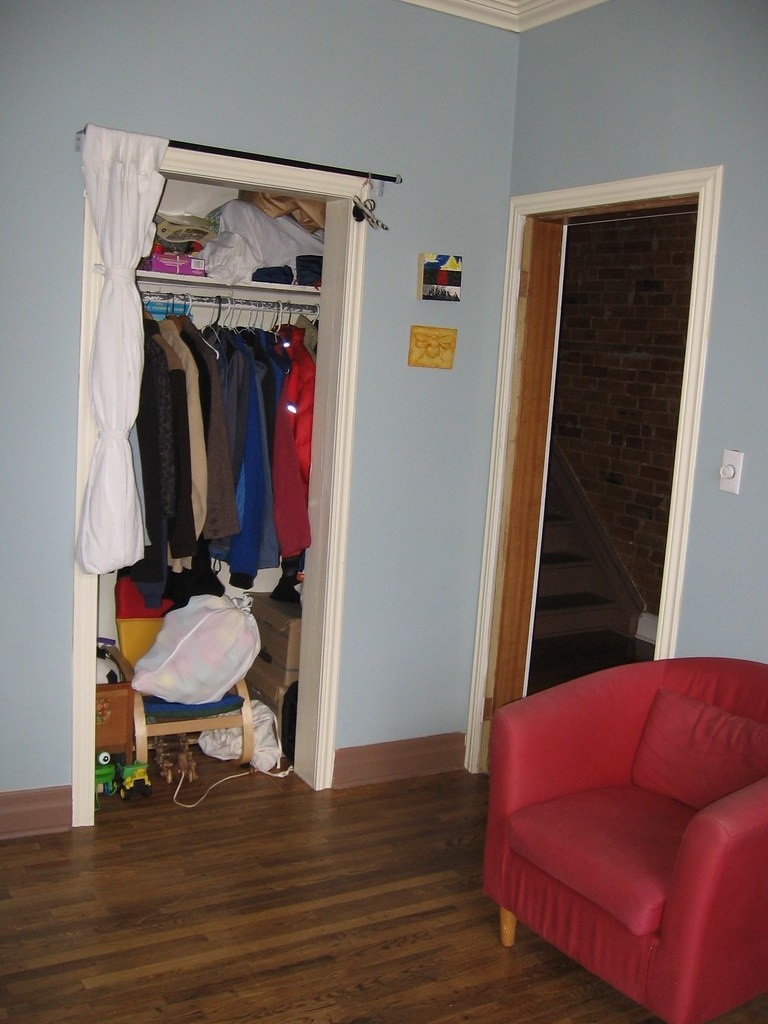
[244,591,302,757]
[142,252,205,276]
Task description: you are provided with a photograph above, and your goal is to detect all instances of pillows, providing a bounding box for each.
[630,687,767,809]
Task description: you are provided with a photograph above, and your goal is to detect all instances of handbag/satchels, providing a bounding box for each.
[131,594,262,705]
[197,699,282,772]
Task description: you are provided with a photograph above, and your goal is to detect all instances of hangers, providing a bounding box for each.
[143,290,320,374]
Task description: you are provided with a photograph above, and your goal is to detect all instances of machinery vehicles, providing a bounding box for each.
[113,759,153,801]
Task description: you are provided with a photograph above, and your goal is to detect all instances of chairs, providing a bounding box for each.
[481,657,768,1024]
[114,574,255,770]
